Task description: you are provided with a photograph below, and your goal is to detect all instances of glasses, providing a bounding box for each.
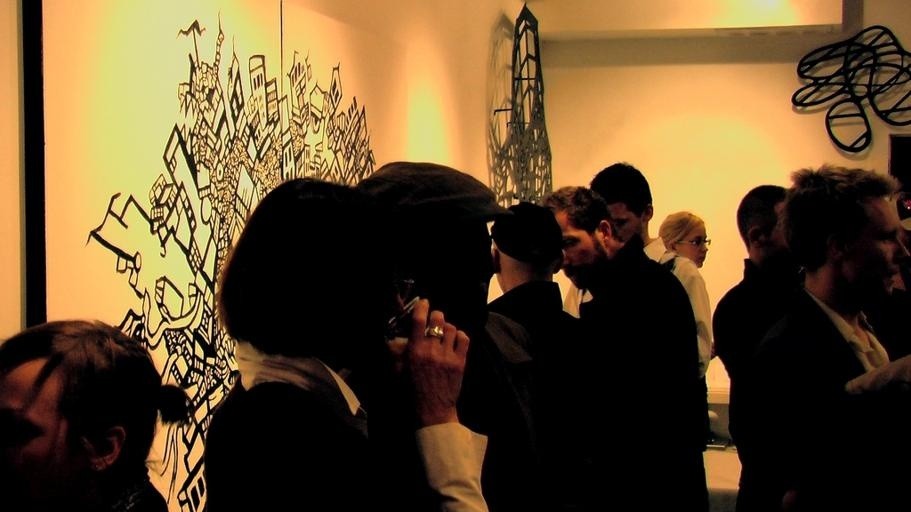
[680,239,711,246]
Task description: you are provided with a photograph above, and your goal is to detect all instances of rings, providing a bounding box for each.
[425,324,445,341]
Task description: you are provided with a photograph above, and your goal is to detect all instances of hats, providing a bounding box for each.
[491,202,564,267]
[356,159,511,236]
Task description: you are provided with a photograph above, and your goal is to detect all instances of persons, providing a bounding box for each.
[0,321,186,509]
[564,164,714,381]
[199,178,488,510]
[546,188,708,509]
[712,186,798,510]
[457,201,613,512]
[659,213,707,267]
[358,164,528,512]
[760,168,911,510]
